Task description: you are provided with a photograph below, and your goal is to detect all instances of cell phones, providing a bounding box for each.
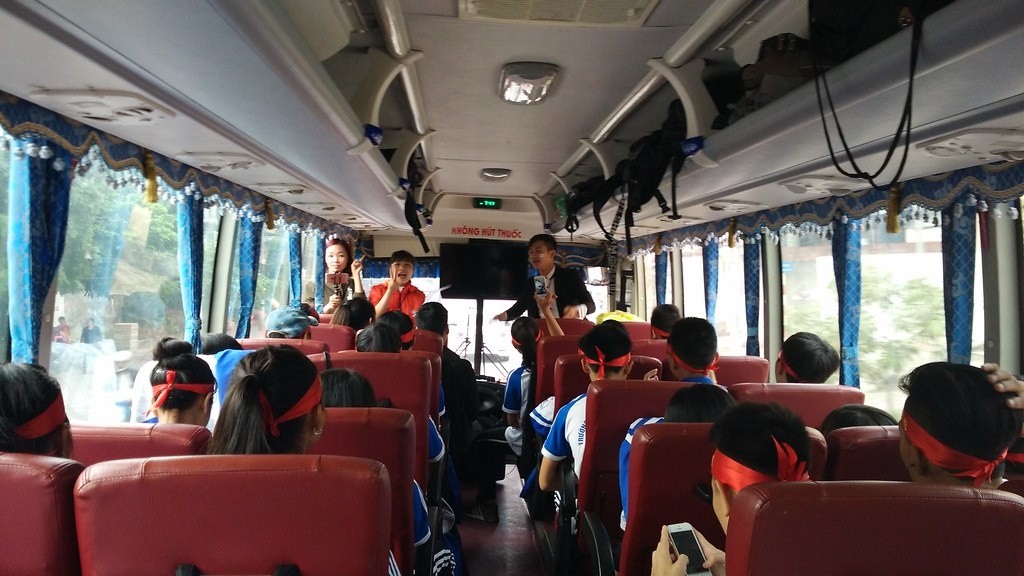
[325,273,349,284]
[534,275,547,297]
[668,523,713,576]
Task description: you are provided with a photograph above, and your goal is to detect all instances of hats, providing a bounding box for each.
[264,304,320,340]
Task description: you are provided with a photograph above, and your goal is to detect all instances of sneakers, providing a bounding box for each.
[466,497,500,523]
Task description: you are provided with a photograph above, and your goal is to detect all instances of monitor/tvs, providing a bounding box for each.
[440,242,541,301]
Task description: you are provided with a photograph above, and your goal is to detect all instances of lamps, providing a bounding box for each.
[480,167,513,184]
[497,62,561,108]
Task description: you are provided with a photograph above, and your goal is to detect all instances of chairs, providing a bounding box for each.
[337,348,453,454]
[579,420,829,576]
[0,453,82,576]
[236,336,330,355]
[305,350,452,503]
[65,422,216,469]
[305,408,440,576]
[729,381,866,430]
[535,317,596,336]
[309,322,355,354]
[554,352,663,423]
[826,422,911,481]
[412,329,445,355]
[725,480,1024,576]
[318,314,334,324]
[630,338,668,364]
[556,378,727,560]
[715,355,769,388]
[623,322,654,339]
[534,335,584,405]
[72,454,393,576]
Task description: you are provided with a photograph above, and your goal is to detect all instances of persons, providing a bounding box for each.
[52,317,71,343]
[330,298,375,328]
[492,234,596,319]
[539,319,635,530]
[369,250,425,330]
[80,318,102,343]
[377,310,444,416]
[650,304,680,339]
[0,362,73,460]
[502,289,565,457]
[356,324,446,462]
[897,362,1024,497]
[265,303,319,339]
[775,332,838,383]
[643,318,729,392]
[140,332,463,576]
[650,401,810,576]
[617,385,736,533]
[417,303,481,431]
[302,238,365,314]
[820,404,897,438]
